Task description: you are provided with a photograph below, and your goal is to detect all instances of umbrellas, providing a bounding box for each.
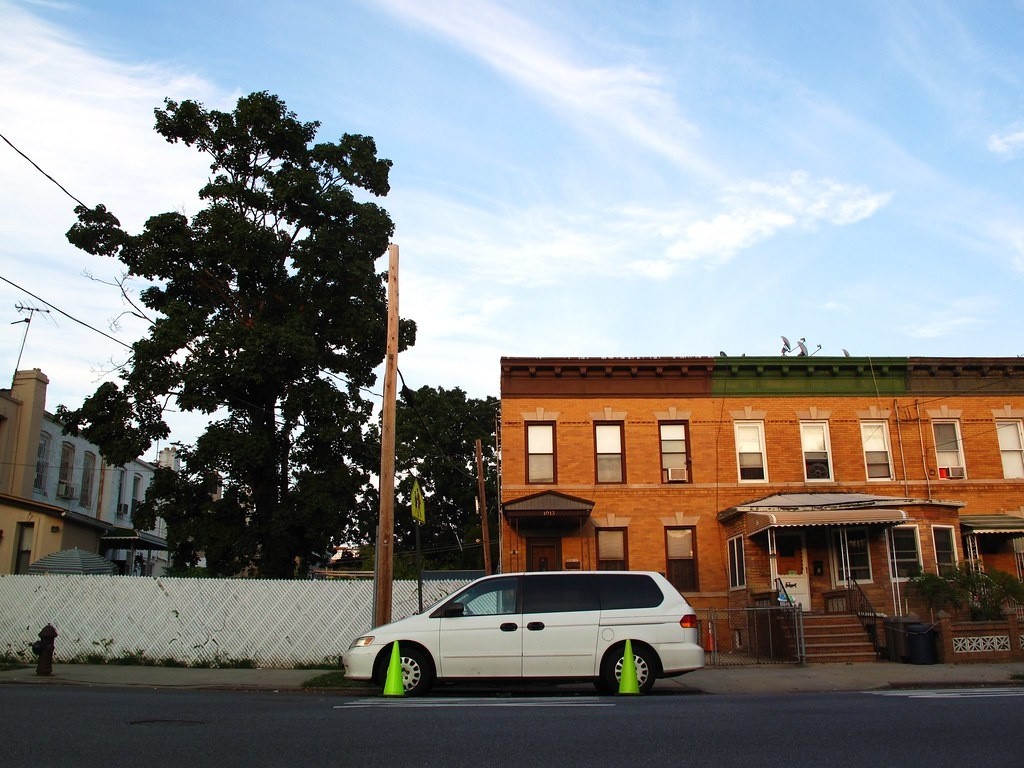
[28,546,120,575]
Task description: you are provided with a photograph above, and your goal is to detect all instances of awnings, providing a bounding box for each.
[103,528,177,551]
[746,509,908,537]
[958,514,1024,535]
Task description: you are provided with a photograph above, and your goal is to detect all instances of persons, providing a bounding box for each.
[291,555,309,579]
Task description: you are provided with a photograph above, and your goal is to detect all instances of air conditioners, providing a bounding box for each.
[117,503,128,515]
[668,468,686,481]
[58,484,74,498]
[946,466,965,479]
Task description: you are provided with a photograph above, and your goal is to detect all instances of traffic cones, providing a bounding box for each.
[380,639,410,698]
[704,620,720,653]
[618,638,646,695]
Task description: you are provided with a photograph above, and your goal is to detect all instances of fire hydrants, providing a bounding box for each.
[32,624,58,676]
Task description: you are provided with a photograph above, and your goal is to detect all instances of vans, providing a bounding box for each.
[342,570,708,696]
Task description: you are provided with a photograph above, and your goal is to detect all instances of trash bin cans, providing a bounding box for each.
[883,616,940,665]
